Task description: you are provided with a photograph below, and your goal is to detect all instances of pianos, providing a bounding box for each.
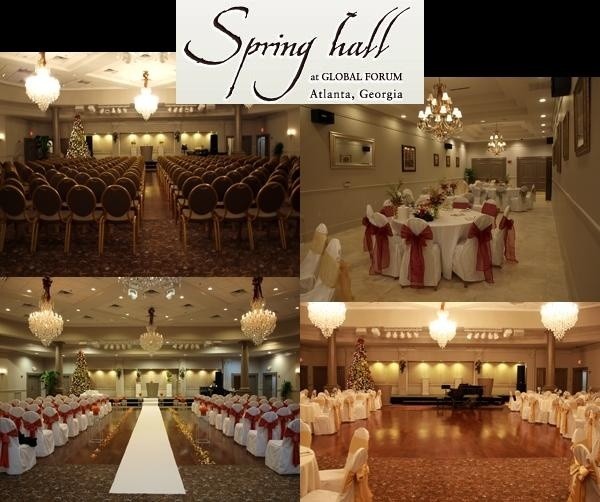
[187,149,208,156]
[448,383,484,396]
[199,387,223,395]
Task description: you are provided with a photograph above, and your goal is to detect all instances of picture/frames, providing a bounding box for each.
[552,77,591,175]
[401,144,416,172]
[456,156,459,167]
[434,153,439,166]
[446,156,450,167]
[329,130,376,169]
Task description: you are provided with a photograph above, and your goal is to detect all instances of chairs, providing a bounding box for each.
[300,387,383,502]
[0,393,113,476]
[191,394,300,475]
[300,180,536,302]
[508,390,600,502]
[0,154,300,257]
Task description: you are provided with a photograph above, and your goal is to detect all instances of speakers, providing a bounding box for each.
[86,136,92,155]
[311,109,334,125]
[446,143,453,149]
[551,77,571,97]
[517,365,524,393]
[210,135,218,153]
[215,372,222,384]
[547,137,553,144]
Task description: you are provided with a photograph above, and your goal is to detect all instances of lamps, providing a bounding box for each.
[240,276,278,347]
[487,123,507,156]
[306,302,346,339]
[428,302,457,350]
[539,301,580,342]
[135,70,159,121]
[139,307,164,357]
[114,277,181,300]
[416,77,464,143]
[28,277,64,347]
[25,52,61,112]
